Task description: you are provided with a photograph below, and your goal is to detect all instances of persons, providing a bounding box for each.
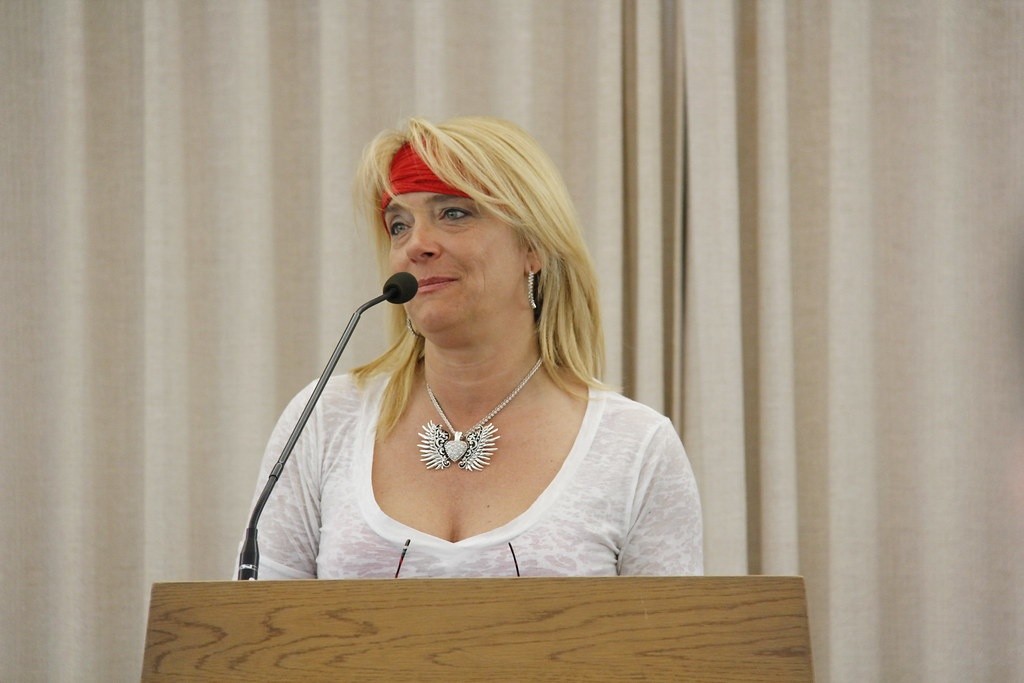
[234,115,704,581]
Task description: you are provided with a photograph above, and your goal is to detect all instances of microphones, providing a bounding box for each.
[238,272,418,580]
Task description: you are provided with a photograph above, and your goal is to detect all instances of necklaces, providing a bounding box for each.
[417,355,542,472]
